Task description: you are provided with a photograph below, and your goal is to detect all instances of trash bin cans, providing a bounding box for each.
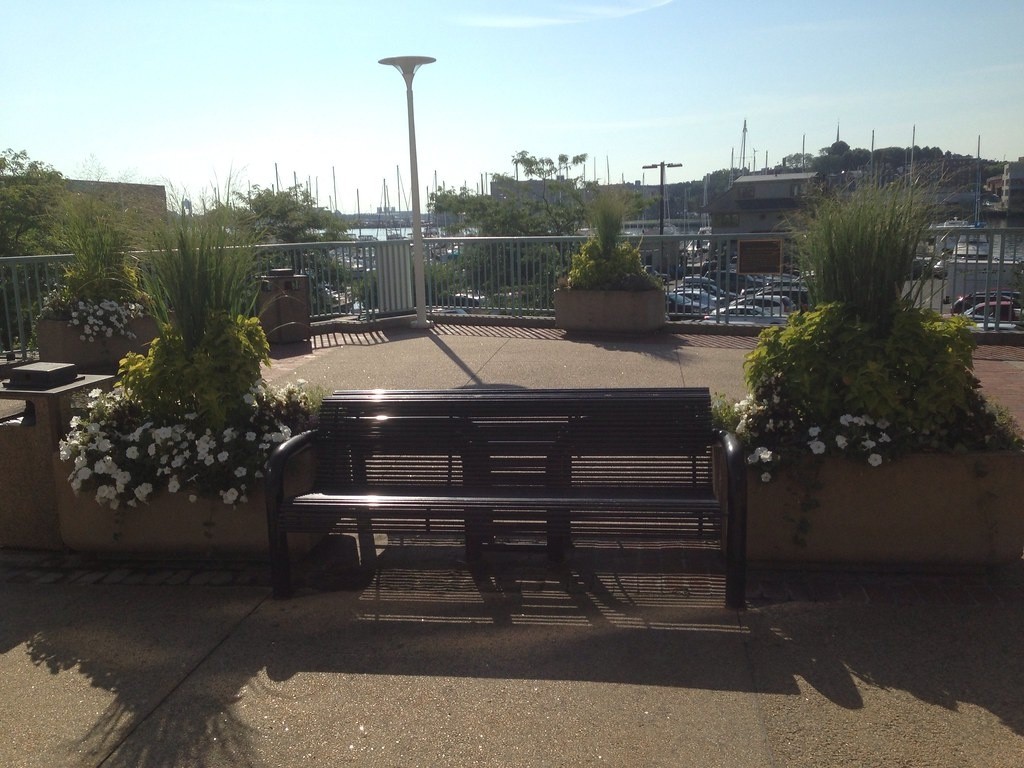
[257,268,309,345]
[0,361,116,552]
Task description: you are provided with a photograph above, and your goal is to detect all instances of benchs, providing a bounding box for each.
[267,387,745,609]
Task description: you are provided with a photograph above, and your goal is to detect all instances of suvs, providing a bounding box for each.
[940,289,1024,331]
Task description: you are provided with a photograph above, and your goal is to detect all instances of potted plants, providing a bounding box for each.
[554,189,667,334]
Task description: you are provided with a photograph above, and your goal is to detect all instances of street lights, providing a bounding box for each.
[641,160,683,262]
[378,54,439,330]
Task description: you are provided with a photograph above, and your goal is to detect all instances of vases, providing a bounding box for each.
[38,312,177,367]
[56,443,322,552]
[743,450,1024,571]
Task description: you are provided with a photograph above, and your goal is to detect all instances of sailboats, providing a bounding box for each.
[952,135,990,260]
[570,155,712,256]
[270,161,489,313]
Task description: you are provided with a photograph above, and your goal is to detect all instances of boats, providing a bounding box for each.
[929,218,969,254]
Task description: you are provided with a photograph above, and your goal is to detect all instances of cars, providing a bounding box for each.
[665,267,814,325]
[652,270,672,283]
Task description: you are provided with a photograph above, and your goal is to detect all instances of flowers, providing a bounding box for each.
[712,166,1024,548]
[26,170,155,357]
[59,173,325,541]
